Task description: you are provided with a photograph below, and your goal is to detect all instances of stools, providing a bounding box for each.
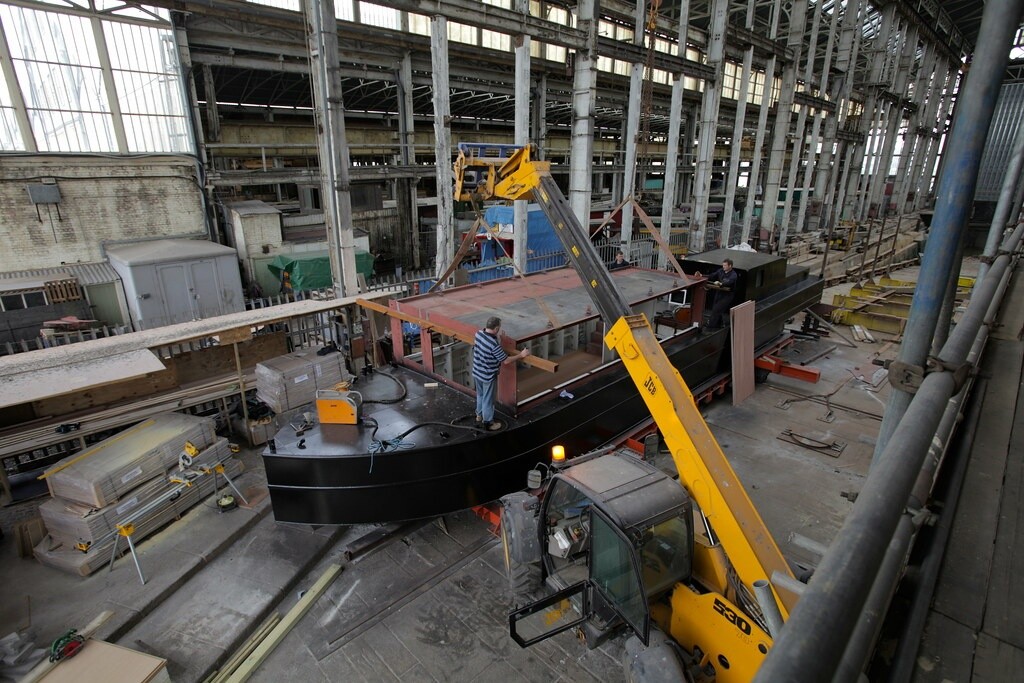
[591,545,640,580]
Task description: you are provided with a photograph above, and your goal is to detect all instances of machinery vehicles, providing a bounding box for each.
[453,142,815,682]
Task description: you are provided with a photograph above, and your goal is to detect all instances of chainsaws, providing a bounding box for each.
[169,439,211,486]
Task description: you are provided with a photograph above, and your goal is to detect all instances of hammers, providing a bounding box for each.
[289,423,304,437]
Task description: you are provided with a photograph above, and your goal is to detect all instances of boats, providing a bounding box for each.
[262,246,825,526]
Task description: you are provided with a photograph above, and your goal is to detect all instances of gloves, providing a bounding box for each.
[694,271,703,277]
[713,281,722,286]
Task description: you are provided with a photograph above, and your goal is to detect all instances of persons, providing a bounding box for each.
[471,317,530,429]
[372,249,383,282]
[769,224,780,256]
[612,251,629,269]
[751,224,761,251]
[695,259,737,331]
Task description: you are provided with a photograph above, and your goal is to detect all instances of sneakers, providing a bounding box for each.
[476,416,483,422]
[482,420,501,432]
